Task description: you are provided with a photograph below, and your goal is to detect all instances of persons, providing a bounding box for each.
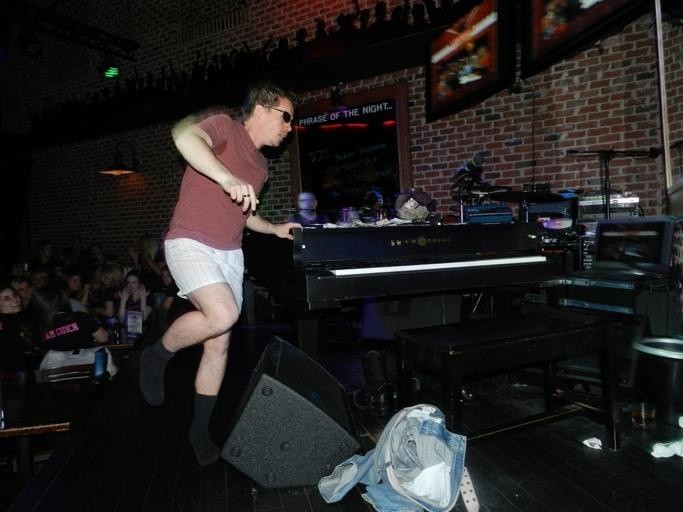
[362,191,384,217]
[288,192,330,225]
[138,84,304,466]
[1,241,171,371]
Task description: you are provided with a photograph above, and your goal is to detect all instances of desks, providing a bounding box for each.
[391,310,623,458]
[0,325,145,512]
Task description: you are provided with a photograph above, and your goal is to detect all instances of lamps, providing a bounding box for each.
[99,36,138,79]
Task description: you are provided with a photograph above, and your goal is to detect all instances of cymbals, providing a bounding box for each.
[489,190,563,204]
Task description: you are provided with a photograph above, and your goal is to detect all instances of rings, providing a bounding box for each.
[243,193,250,198]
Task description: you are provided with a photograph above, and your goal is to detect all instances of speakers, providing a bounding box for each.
[220,335,362,490]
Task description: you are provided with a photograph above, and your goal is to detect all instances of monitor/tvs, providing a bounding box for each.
[425,0,518,123]
[521,0,652,79]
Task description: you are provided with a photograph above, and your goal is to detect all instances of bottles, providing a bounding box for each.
[94,348,106,377]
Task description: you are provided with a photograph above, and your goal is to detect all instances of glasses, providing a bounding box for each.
[256,103,295,126]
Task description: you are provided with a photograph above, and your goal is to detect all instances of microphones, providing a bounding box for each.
[453,154,485,178]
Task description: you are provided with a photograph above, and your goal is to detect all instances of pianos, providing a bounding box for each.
[242,220,555,363]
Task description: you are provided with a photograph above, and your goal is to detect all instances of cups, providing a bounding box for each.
[631,402,657,435]
